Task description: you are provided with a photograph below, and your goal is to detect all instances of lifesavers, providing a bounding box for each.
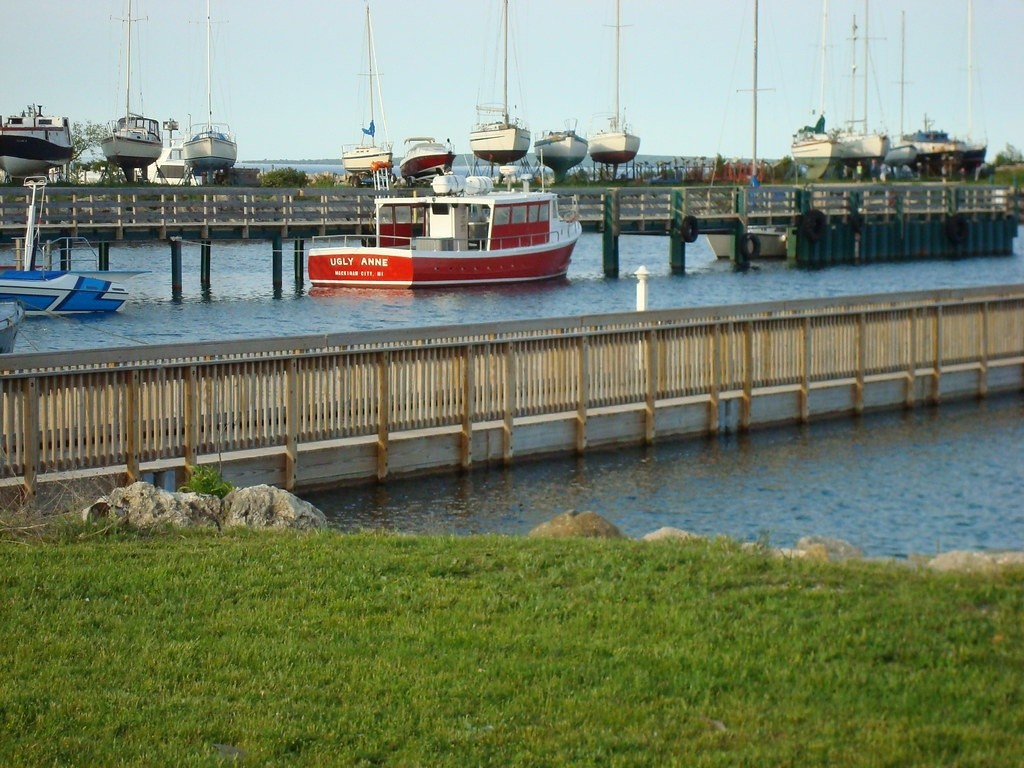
[851,214,865,232]
[683,215,698,242]
[803,208,827,242]
[741,232,761,259]
[947,215,973,242]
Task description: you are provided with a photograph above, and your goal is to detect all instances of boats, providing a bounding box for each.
[900,113,988,176]
[0,176,153,318]
[0,104,75,177]
[882,145,918,169]
[307,149,583,291]
[534,118,588,171]
[399,137,457,182]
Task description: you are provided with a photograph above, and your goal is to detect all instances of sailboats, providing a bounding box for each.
[181,0,238,172]
[791,0,846,180]
[469,0,531,163]
[340,1,393,173]
[704,0,788,261]
[100,0,163,167]
[587,0,641,165]
[837,0,891,175]
